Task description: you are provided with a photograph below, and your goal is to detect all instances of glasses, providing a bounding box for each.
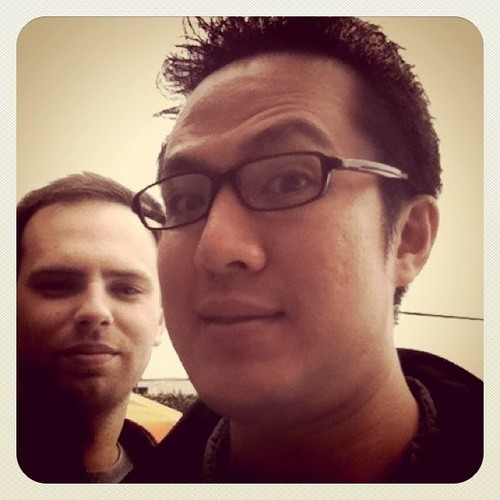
[131,151,422,231]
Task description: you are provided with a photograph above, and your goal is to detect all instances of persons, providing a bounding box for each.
[17,174,183,483]
[129,16,484,483]
